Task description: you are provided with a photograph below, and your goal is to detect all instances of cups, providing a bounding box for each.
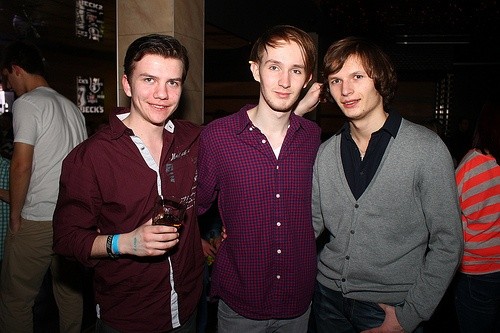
[152,199,187,230]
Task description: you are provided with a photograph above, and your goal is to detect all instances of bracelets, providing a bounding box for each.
[112,234,121,255]
[106,235,114,258]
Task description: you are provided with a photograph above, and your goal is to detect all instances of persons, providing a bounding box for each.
[0,49,89,333]
[218,35,463,333]
[452,96,500,333]
[193,25,324,333]
[50,34,326,333]
[0,155,15,263]
